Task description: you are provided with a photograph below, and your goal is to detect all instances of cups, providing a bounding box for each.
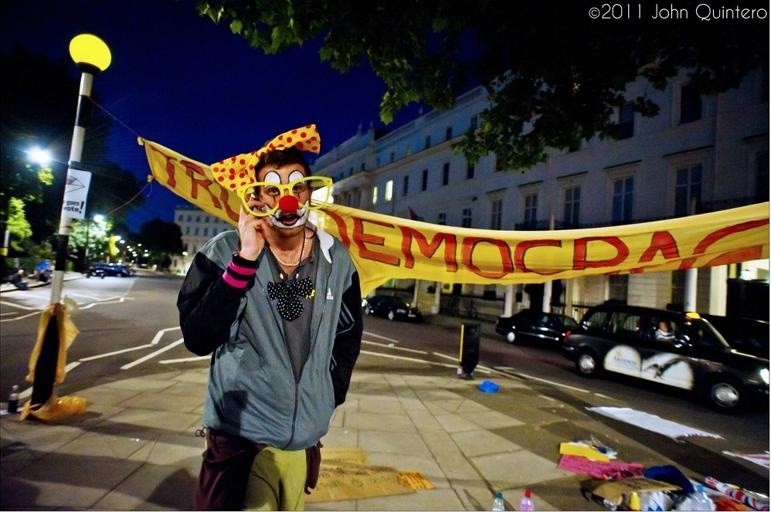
[7,384,20,414]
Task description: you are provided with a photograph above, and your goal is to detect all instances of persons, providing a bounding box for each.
[654,320,678,343]
[174,143,368,510]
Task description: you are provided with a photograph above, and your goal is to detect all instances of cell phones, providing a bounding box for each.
[251,193,266,213]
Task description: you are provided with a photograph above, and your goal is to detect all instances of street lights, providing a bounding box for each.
[0,145,49,282]
[84,213,104,273]
[26,31,111,421]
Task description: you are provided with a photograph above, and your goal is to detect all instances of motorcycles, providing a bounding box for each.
[85,266,105,279]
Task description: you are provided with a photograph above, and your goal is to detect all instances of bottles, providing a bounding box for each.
[519,489,534,512]
[492,492,505,512]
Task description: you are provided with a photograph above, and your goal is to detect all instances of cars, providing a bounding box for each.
[92,264,135,278]
[362,296,420,323]
[495,301,769,416]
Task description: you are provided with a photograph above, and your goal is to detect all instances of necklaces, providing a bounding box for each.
[268,238,306,267]
